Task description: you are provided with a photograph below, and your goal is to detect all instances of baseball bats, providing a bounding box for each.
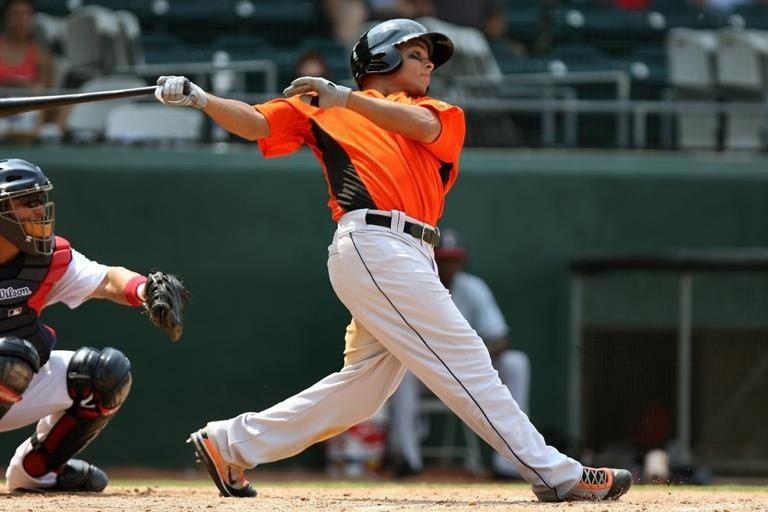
[0,78,190,117]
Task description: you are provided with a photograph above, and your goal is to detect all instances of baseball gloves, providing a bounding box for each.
[140,271,195,341]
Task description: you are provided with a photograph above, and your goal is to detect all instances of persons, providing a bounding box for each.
[294,1,520,145]
[152,16,634,498]
[375,225,531,481]
[0,2,77,143]
[0,158,194,493]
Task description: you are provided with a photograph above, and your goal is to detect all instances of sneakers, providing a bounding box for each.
[493,468,525,483]
[569,465,633,500]
[394,458,419,481]
[186,426,259,498]
[39,457,109,492]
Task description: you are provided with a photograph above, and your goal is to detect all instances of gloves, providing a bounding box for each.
[283,76,352,110]
[155,76,208,110]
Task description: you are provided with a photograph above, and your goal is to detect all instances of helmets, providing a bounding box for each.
[350,18,455,91]
[0,159,56,257]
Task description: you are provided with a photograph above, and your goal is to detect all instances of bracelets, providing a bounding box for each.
[124,274,149,306]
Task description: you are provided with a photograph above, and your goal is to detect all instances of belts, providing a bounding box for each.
[366,212,441,246]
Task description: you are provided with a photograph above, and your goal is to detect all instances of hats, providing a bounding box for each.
[434,226,467,258]
